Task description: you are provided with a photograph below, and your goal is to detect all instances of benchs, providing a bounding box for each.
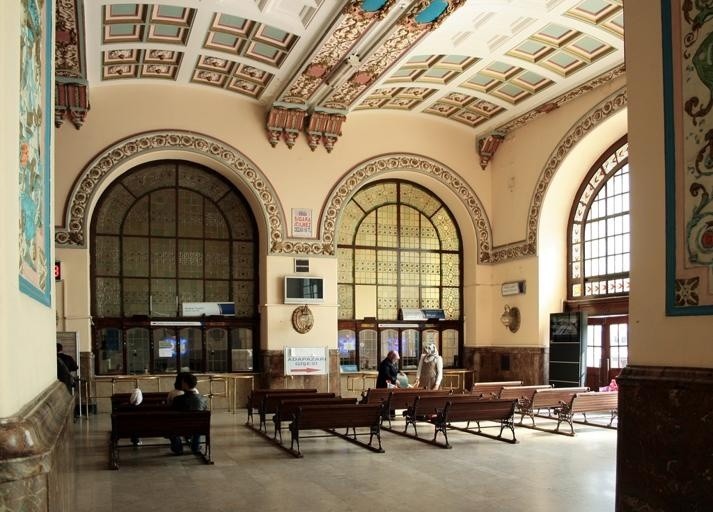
[107,393,214,471]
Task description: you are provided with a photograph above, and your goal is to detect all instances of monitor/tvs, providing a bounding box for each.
[283,275,325,305]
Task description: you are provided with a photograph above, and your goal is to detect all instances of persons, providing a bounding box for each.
[167,372,200,444]
[57,344,78,395]
[165,372,208,456]
[416,343,443,422]
[376,350,405,420]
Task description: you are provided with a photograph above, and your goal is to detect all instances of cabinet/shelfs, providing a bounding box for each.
[548,311,587,387]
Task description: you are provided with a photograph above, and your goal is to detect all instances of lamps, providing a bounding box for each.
[500,305,520,333]
[292,305,314,334]
